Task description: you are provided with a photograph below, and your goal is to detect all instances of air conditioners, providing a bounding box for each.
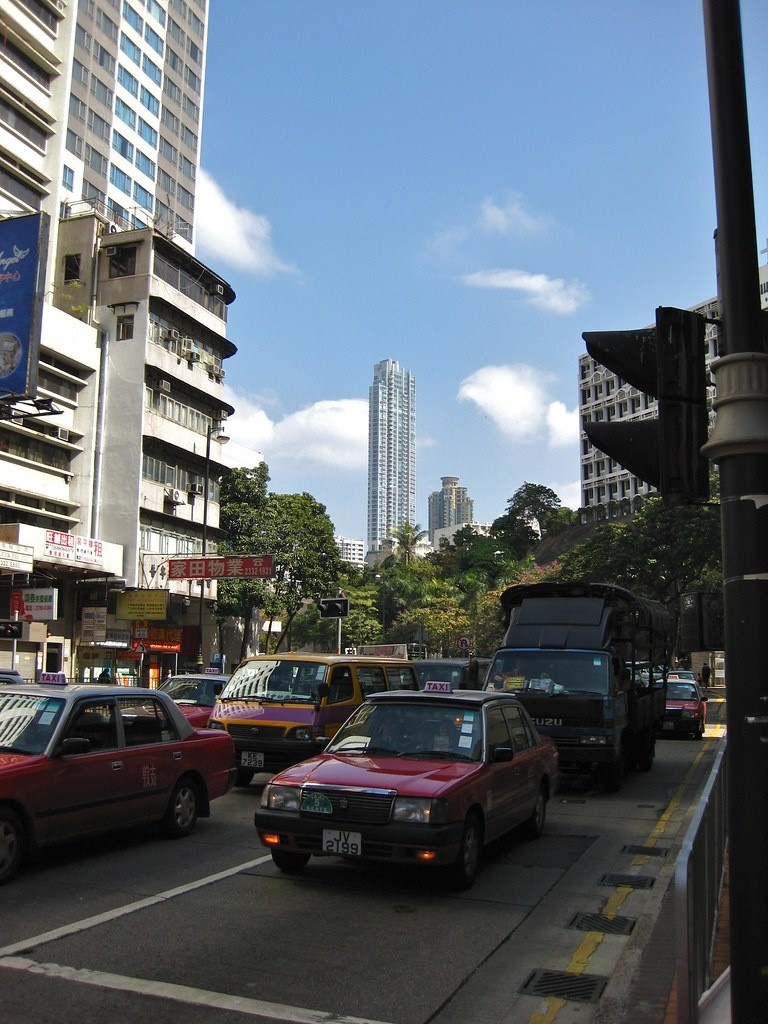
[209,284,223,295]
[187,483,202,494]
[189,352,200,362]
[51,427,69,441]
[10,410,23,425]
[167,329,179,340]
[106,221,118,233]
[153,380,171,392]
[216,369,226,378]
[207,364,219,374]
[217,409,228,421]
[169,489,183,503]
[105,247,117,256]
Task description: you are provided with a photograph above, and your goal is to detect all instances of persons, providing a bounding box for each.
[641,670,647,687]
[505,658,530,681]
[441,720,457,748]
[164,669,173,689]
[329,669,337,702]
[98,669,110,684]
[375,718,404,746]
[698,663,710,689]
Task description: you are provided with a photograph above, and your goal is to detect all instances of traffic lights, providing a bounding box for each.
[317,599,349,619]
[579,308,710,508]
[0,621,22,638]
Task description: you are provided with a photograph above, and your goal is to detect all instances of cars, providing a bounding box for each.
[110,673,235,728]
[628,661,709,739]
[0,669,239,887]
[254,680,565,887]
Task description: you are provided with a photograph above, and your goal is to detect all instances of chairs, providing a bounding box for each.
[328,676,352,702]
[74,712,110,742]
[126,716,162,746]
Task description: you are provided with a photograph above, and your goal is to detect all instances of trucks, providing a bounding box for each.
[478,580,670,793]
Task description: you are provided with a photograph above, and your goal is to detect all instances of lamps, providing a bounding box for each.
[183,596,191,606]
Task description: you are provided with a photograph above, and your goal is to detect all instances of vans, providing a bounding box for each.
[207,650,505,785]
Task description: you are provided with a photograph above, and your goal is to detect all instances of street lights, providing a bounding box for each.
[196,426,230,670]
